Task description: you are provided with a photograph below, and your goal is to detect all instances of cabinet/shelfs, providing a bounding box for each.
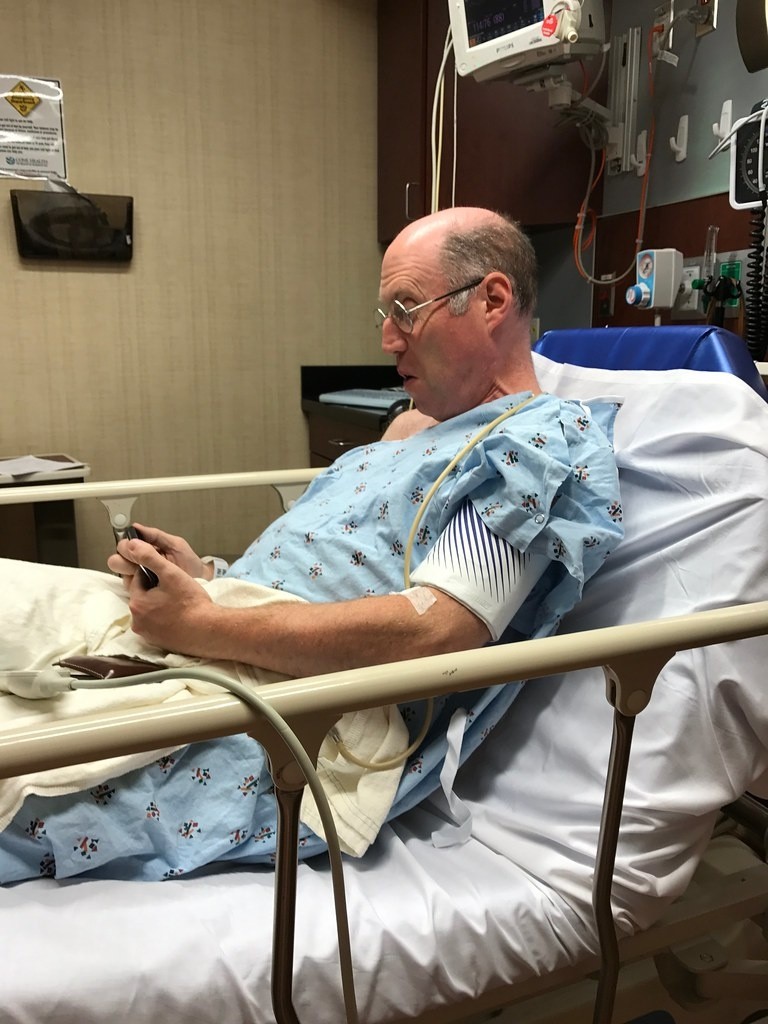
[301,399,388,468]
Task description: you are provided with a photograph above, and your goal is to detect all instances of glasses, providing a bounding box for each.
[373,278,484,334]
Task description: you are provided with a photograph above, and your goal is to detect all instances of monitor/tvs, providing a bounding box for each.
[448,0,606,85]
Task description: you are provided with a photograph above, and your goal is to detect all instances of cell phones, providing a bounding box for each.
[126,526,159,587]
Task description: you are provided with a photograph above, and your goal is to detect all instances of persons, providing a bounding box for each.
[0,206,625,886]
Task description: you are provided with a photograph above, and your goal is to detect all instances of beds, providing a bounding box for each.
[0,328,768,1024]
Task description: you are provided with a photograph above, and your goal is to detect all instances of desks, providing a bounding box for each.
[0,454,91,569]
